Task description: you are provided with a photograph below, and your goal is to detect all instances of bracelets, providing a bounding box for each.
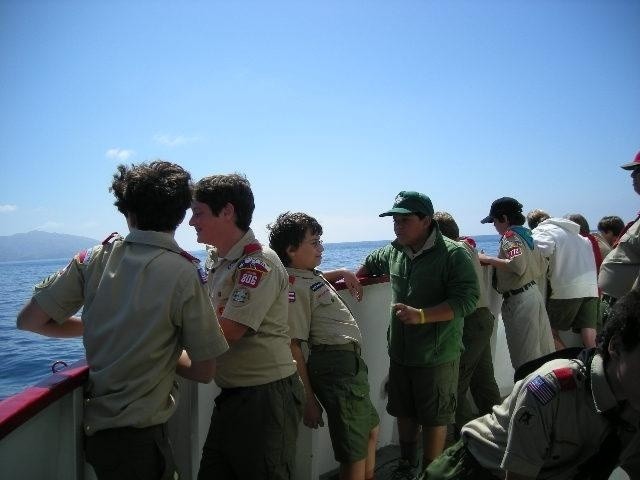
[418,309,425,324]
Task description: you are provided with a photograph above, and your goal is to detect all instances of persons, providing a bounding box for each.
[598,151,640,341]
[433,211,501,439]
[16,161,229,480]
[355,192,481,480]
[417,290,640,479]
[527,209,599,348]
[562,214,603,273]
[189,172,306,479]
[268,211,380,479]
[598,216,625,244]
[480,196,556,372]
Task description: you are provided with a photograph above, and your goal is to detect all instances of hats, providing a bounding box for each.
[622,151,640,170]
[379,191,434,216]
[481,197,523,223]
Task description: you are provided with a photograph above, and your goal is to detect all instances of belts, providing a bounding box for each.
[312,343,361,354]
[503,280,535,296]
[603,294,618,302]
